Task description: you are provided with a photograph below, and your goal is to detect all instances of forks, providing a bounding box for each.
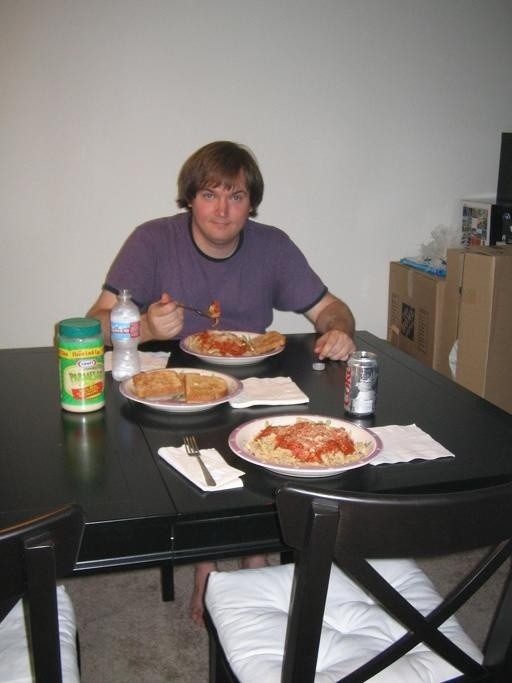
[155,301,220,319]
[183,436,216,486]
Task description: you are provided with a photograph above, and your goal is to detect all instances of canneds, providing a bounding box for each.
[344,351,379,416]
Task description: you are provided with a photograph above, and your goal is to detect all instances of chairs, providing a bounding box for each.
[0,502,101,683]
[203,486,512,683]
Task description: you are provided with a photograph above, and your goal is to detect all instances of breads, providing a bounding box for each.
[133,370,228,404]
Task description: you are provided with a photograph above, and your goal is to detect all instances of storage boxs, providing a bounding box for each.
[440,245,512,415]
[387,262,444,369]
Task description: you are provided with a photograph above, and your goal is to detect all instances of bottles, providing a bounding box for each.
[111,290,140,380]
[57,317,106,414]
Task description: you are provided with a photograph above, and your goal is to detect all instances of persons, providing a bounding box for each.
[83,137,356,631]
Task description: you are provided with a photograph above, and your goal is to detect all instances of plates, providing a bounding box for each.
[229,413,383,477]
[180,332,285,365]
[120,368,243,413]
